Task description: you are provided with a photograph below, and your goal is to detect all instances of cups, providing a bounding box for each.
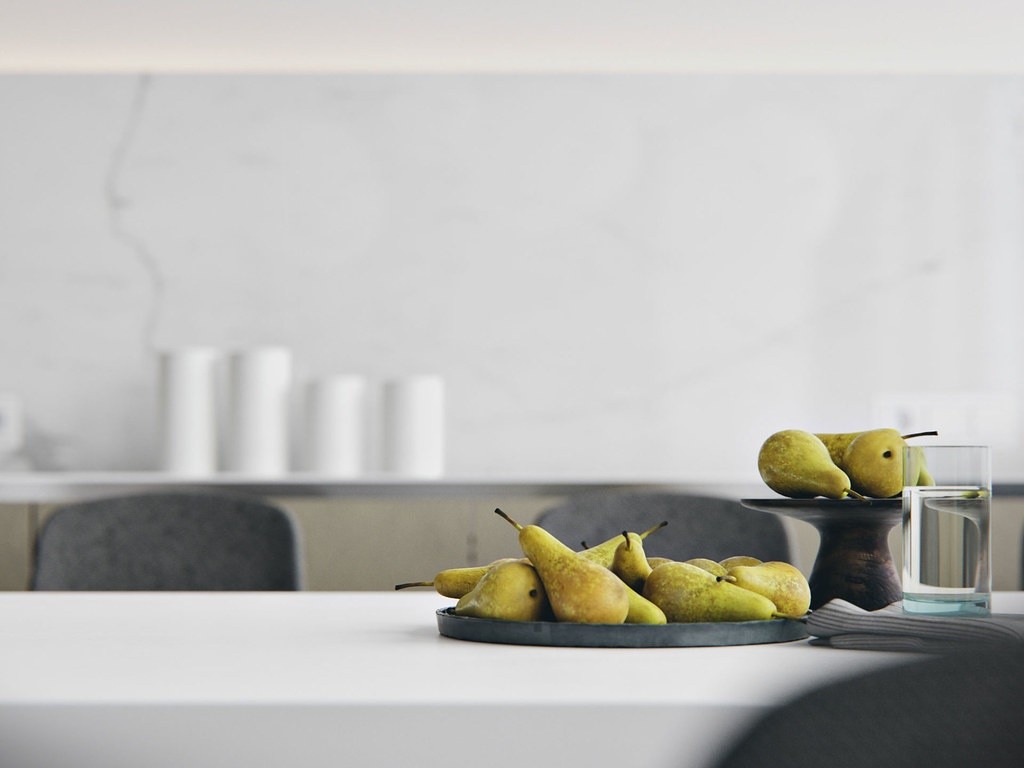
[229,346,290,472]
[380,375,443,477]
[902,446,991,619]
[161,347,219,475]
[305,375,370,473]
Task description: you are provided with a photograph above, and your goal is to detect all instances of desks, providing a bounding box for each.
[2,589,1024,768]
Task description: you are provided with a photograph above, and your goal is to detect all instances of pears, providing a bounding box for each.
[758,428,940,505]
[391,506,811,623]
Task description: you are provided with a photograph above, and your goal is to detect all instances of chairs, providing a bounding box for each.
[534,493,797,566]
[720,643,1024,768]
[30,494,303,592]
